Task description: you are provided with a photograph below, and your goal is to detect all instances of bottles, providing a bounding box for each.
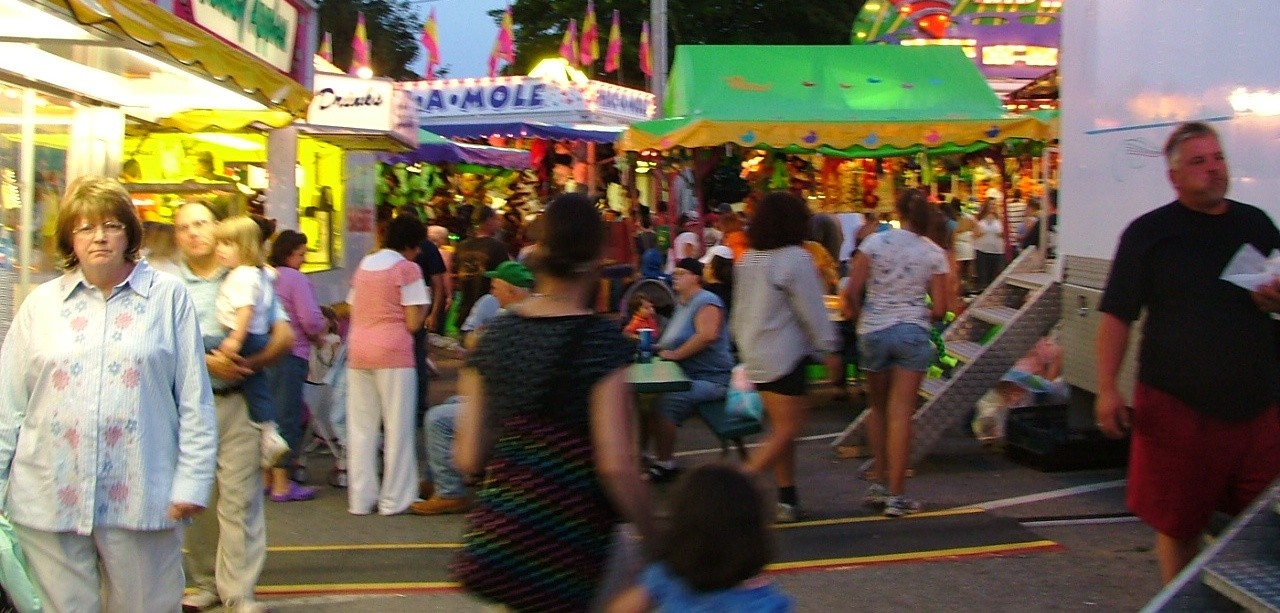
[445,291,463,331]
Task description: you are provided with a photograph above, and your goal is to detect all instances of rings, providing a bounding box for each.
[1097,424,1103,430]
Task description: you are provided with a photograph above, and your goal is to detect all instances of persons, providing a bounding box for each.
[122,158,159,222]
[202,215,290,470]
[410,261,535,515]
[449,193,660,613]
[433,205,525,328]
[640,259,735,484]
[726,192,841,522]
[838,188,949,516]
[1096,122,1280,587]
[398,206,449,427]
[1006,188,1057,261]
[0,175,219,613]
[344,213,432,516]
[624,292,662,337]
[263,229,328,501]
[942,197,1005,285]
[632,200,746,284]
[173,202,295,613]
[605,463,795,613]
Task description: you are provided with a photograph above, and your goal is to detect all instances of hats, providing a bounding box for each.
[677,257,703,276]
[482,261,536,286]
[699,245,736,264]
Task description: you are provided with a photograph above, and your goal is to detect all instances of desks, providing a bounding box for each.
[621,354,692,473]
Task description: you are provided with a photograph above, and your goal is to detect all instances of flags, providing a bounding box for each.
[319,12,653,80]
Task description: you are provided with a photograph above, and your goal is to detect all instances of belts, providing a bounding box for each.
[213,386,240,396]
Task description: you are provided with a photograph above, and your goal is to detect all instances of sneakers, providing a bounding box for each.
[884,497,927,516]
[775,498,809,522]
[862,487,890,502]
[259,424,289,470]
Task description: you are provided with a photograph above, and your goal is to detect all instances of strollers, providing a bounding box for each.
[286,342,384,490]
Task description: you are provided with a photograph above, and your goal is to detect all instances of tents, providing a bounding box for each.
[619,44,1052,259]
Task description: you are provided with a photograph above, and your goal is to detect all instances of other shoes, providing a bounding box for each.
[426,359,440,379]
[320,445,337,454]
[223,597,269,613]
[182,590,219,611]
[304,441,318,452]
[327,470,346,486]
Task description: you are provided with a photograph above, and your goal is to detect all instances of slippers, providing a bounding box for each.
[640,464,680,480]
[268,482,316,501]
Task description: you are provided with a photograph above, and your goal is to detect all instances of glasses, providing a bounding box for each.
[72,222,127,235]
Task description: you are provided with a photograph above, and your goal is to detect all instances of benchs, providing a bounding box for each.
[693,399,765,461]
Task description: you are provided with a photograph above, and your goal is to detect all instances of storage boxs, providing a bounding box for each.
[1002,406,1132,474]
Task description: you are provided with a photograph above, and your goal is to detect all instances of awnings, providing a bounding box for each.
[380,129,531,169]
[0,0,316,131]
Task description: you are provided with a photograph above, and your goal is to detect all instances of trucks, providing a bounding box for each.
[1047,0,1280,440]
[114,106,345,275]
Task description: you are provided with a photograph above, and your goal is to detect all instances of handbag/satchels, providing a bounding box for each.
[721,363,762,422]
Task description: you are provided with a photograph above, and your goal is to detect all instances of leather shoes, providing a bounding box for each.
[418,477,434,498]
[411,493,469,514]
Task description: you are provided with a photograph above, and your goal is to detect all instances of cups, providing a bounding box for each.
[636,327,655,359]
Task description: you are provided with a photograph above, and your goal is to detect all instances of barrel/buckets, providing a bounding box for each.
[818,295,848,352]
